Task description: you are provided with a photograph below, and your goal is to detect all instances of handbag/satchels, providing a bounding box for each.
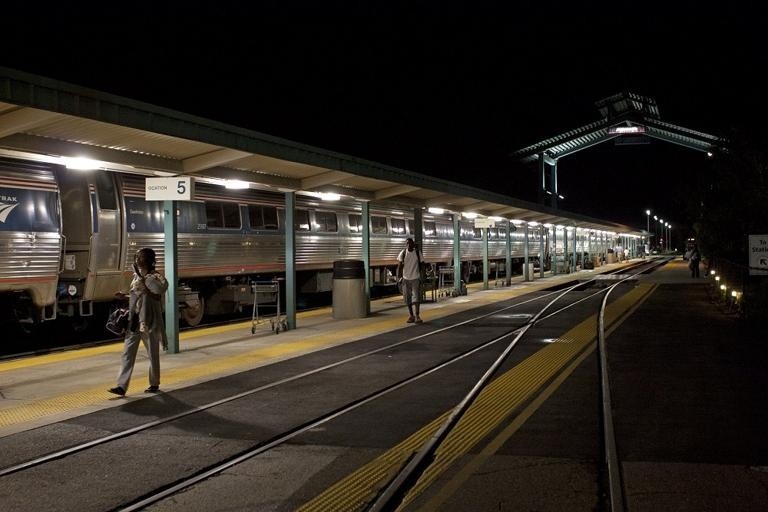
[105,307,129,337]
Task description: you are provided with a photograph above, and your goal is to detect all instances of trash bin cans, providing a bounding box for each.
[332,259,366,318]
[522,263,534,281]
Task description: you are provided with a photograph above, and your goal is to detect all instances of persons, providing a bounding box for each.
[106,248,168,395]
[689,249,701,278]
[623,246,630,263]
[614,242,623,264]
[396,238,426,323]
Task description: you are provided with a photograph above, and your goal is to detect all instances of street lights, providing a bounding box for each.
[645,208,672,255]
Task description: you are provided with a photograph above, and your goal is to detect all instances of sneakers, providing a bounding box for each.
[144,385,160,393]
[407,316,423,323]
[107,387,126,397]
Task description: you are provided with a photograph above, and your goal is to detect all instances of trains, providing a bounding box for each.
[0,150,647,356]
